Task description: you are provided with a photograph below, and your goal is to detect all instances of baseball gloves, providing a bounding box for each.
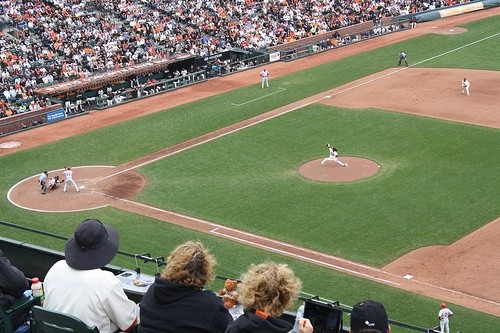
[326,143,330,148]
[60,180,64,184]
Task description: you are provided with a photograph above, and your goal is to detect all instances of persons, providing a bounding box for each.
[41,218,138,333]
[460,77,471,96]
[320,143,348,167]
[0,0,474,136]
[348,299,391,333]
[62,166,80,193]
[437,303,453,333]
[224,261,314,333]
[260,68,270,88]
[137,241,233,333]
[0,249,33,331]
[48,176,64,190]
[398,51,409,68]
[38,171,53,195]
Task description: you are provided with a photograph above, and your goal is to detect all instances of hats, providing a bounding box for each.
[441,304,446,308]
[350,300,388,332]
[65,219,120,271]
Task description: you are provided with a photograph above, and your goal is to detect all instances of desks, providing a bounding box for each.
[116,270,156,296]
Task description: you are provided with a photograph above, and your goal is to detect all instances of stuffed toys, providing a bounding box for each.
[218,278,240,308]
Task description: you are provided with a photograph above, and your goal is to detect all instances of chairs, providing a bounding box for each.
[0,296,43,333]
[28,304,137,333]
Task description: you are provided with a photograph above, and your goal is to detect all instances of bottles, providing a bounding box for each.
[30,277,45,307]
[291,301,305,333]
